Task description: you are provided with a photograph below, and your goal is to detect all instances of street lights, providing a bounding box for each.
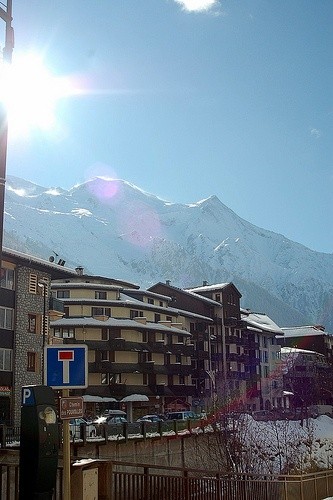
[207,322,220,418]
[34,279,52,384]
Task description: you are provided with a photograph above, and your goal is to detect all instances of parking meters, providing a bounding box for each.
[19,383,60,500]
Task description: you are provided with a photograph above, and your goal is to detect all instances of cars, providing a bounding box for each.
[68,410,310,434]
